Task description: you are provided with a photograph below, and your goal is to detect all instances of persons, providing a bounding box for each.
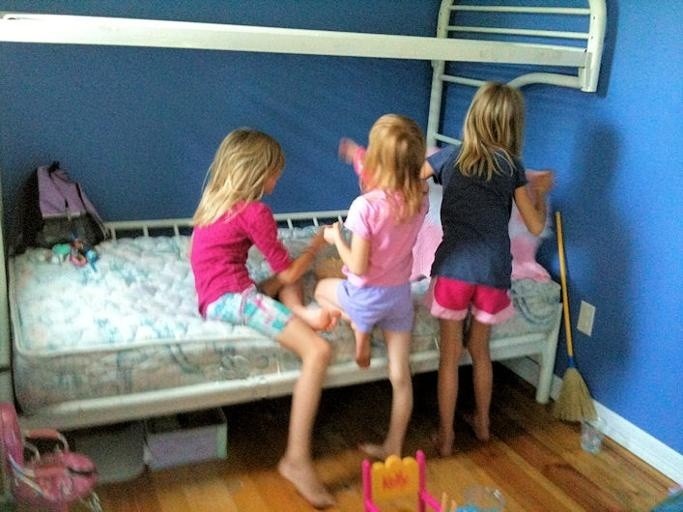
[192,127,341,509]
[420,83,547,455]
[312,114,430,462]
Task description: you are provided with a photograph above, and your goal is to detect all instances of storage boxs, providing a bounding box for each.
[145,408,230,470]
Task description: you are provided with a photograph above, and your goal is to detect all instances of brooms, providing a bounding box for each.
[553,211,596,423]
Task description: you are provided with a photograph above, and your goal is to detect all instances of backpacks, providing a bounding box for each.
[23,161,110,247]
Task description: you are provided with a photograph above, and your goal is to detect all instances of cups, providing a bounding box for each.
[580,415,607,454]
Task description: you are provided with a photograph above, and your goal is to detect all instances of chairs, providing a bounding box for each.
[0,402,104,512]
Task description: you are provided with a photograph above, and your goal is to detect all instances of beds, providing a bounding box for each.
[0,0,609,437]
